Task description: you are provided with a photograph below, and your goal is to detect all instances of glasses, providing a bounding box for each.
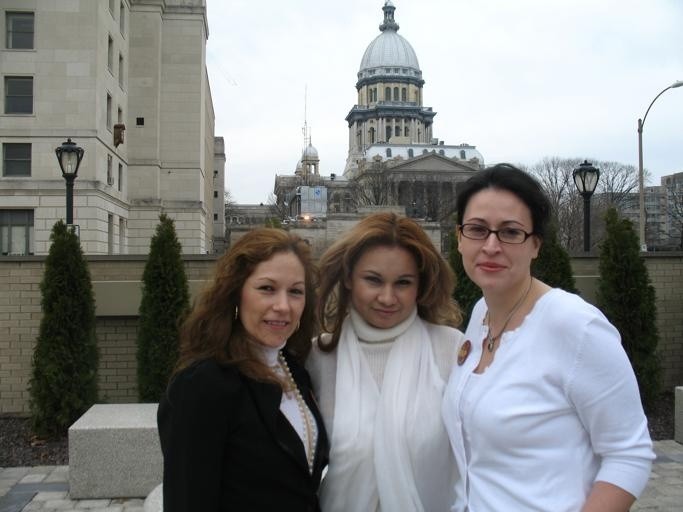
[457,222,534,246]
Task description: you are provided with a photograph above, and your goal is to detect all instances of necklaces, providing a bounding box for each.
[485,275,533,353]
[278,351,316,476]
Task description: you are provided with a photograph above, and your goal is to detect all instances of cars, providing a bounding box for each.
[311,214,327,222]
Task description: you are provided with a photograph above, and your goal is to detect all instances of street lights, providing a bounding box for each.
[572,158,600,251]
[637,79,683,252]
[55,137,85,246]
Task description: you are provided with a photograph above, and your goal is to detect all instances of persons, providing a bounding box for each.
[304,206,467,511]
[154,224,330,511]
[437,160,656,512]
